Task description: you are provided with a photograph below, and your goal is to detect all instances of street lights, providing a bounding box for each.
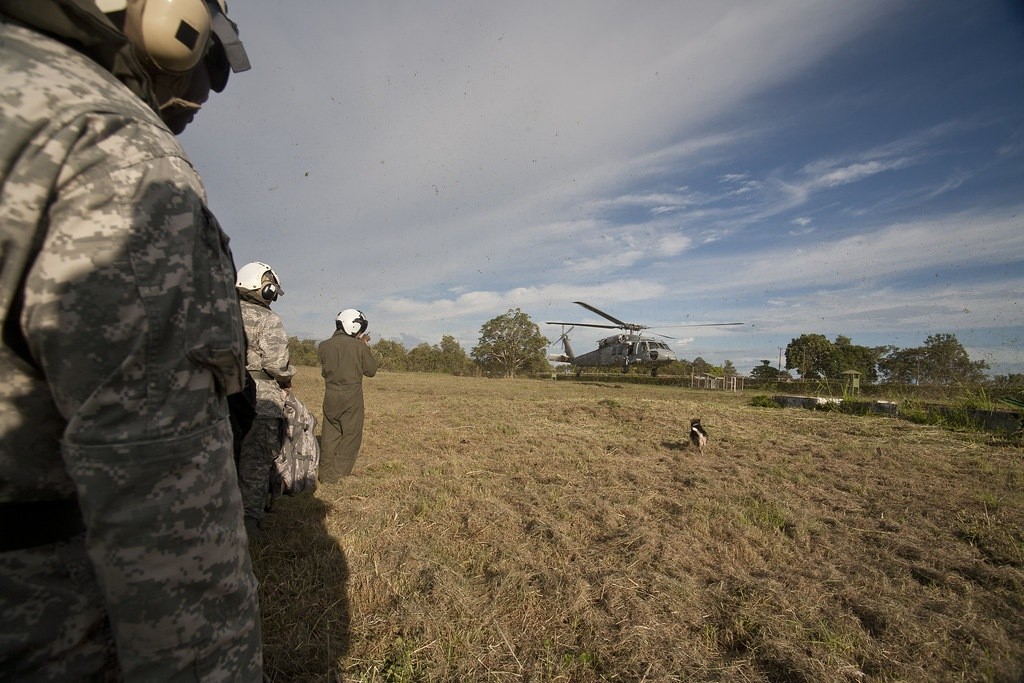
[916,352,927,386]
[690,363,694,374]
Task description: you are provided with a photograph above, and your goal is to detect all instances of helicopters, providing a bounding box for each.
[546,302,744,378]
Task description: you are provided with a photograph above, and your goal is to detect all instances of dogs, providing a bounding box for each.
[689,418,709,456]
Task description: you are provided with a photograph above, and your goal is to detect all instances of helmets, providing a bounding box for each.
[96,0,228,108]
[235,260,280,290]
[335,308,368,337]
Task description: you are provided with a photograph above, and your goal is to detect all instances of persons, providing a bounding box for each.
[0,0,264,683]
[319,309,377,482]
[232,261,298,531]
